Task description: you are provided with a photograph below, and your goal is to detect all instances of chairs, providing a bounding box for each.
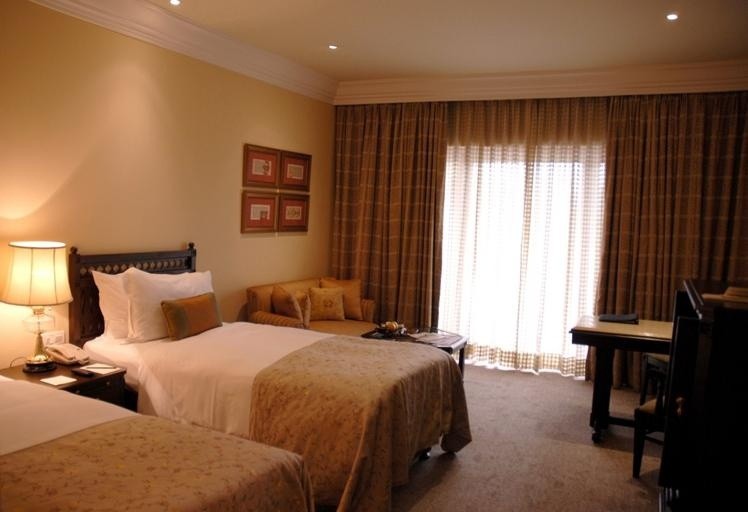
[637,350,670,406]
[634,375,667,477]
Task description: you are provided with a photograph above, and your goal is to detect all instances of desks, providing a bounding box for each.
[568,315,676,443]
[363,321,467,383]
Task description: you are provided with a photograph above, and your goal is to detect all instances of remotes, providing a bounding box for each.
[70,368,95,377]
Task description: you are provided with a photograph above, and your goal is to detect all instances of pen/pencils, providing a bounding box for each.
[88,367,116,369]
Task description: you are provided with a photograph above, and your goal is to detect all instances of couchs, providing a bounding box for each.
[244,277,381,339]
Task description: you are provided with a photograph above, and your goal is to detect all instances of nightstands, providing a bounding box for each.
[1,359,128,405]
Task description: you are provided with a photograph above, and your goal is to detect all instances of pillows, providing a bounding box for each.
[271,276,364,327]
[121,267,218,346]
[159,292,223,341]
[91,272,131,343]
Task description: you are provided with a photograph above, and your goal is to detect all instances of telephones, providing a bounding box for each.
[43,343,90,365]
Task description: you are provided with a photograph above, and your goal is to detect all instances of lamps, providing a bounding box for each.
[1,239,73,372]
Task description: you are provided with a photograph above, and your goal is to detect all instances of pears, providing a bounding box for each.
[389,319,398,331]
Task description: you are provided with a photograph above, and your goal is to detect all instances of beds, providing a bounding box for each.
[68,243,473,511]
[0,373,312,511]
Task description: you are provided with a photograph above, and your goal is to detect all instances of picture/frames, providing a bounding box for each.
[280,151,314,191]
[239,190,278,234]
[280,192,310,233]
[242,138,281,189]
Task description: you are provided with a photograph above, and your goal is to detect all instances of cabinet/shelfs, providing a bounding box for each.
[658,277,748,512]
